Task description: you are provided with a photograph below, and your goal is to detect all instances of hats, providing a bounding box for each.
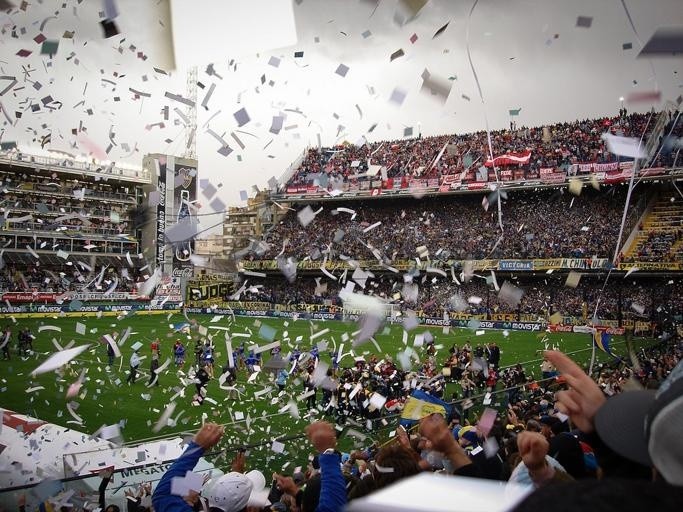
[594,358,683,487]
[208,469,267,512]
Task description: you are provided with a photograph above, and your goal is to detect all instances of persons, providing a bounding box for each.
[1,108,683,511]
